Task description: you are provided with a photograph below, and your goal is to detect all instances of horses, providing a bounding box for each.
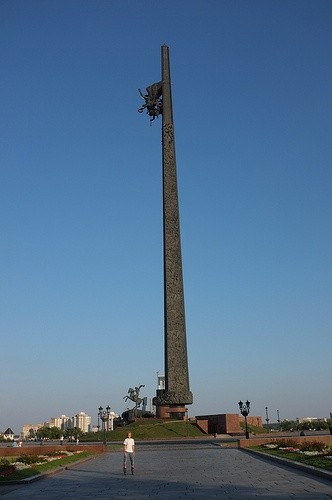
[123,388,144,410]
[138,88,160,121]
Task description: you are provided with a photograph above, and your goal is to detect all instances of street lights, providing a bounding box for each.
[238,399,250,440]
[98,405,111,445]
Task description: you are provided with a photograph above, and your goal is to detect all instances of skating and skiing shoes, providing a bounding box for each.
[131,468,135,475]
[123,468,127,475]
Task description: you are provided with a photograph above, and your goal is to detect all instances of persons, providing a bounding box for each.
[122,431,135,475]
[12,440,23,447]
[131,384,142,402]
